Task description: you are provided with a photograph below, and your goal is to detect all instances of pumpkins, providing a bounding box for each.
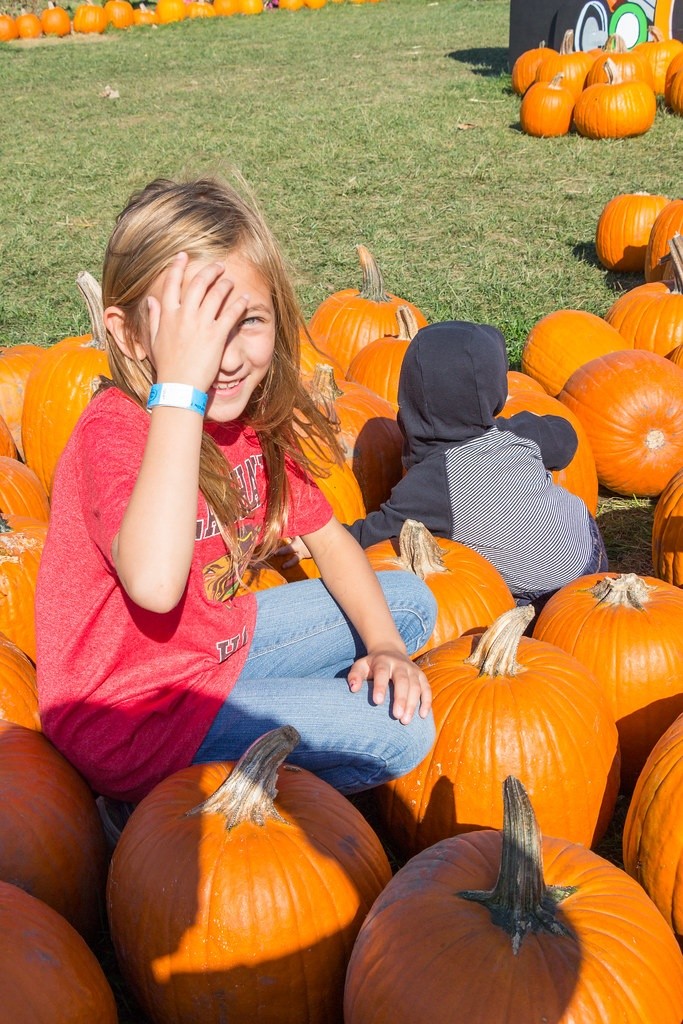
[0,188,683,1023]
[512,25,683,140]
[0,0,378,41]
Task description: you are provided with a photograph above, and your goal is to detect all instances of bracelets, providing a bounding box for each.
[147,383,207,417]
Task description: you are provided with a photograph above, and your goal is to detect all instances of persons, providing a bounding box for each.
[269,320,609,640]
[34,152,436,848]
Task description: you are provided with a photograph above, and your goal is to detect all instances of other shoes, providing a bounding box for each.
[94,796,137,843]
